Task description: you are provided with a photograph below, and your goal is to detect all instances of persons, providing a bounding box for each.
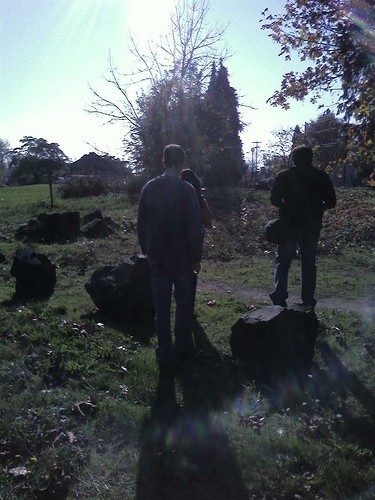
[269,147,337,307]
[137,144,213,359]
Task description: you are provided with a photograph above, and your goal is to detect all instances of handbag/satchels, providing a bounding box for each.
[263,216,291,245]
